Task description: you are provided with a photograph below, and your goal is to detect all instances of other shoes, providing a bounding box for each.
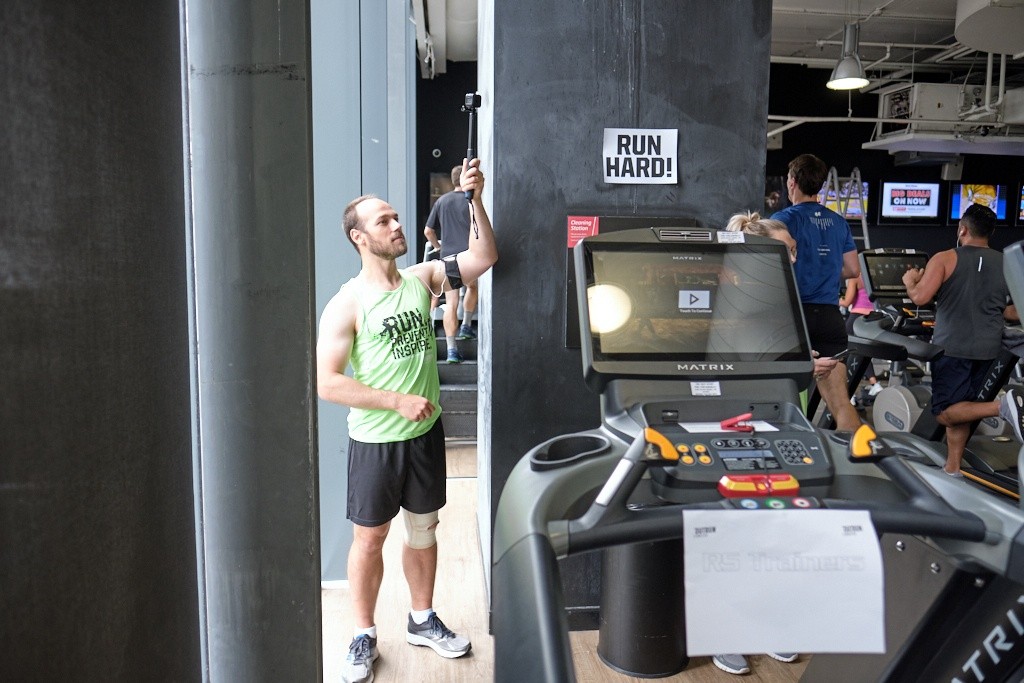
[768,652,799,662]
[712,655,750,674]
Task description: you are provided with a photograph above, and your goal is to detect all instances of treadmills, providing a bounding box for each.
[492,226,1024,683]
[942,348,1022,488]
[811,248,1021,510]
[1002,239,1024,330]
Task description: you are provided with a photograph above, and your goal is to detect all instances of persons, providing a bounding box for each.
[901,202,1024,479]
[838,272,876,337]
[725,209,839,420]
[317,159,498,683]
[769,154,860,432]
[424,166,478,364]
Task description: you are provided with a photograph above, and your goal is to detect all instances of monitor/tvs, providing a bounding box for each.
[573,238,815,381]
[816,178,870,223]
[951,181,1008,224]
[875,178,948,226]
[862,251,931,293]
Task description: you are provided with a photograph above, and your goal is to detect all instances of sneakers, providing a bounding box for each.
[459,323,476,339]
[447,349,464,363]
[340,634,379,683]
[998,389,1024,443]
[406,611,471,658]
[940,464,963,479]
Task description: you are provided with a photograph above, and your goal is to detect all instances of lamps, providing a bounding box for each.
[825,0,871,90]
